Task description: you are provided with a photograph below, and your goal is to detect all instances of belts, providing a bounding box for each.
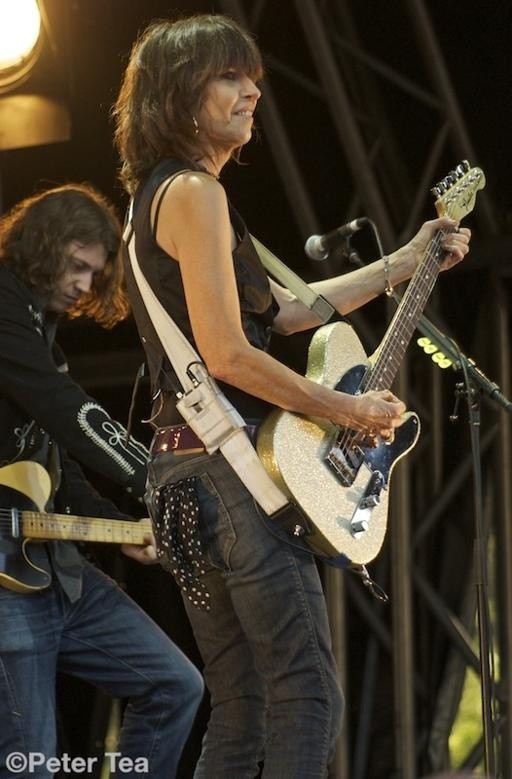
[150,424,261,456]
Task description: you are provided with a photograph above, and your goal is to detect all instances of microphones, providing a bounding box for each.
[304,216,368,261]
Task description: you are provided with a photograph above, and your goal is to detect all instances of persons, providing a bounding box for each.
[0,185,207,779]
[120,15,474,779]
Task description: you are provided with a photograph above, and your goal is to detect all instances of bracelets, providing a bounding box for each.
[382,252,394,299]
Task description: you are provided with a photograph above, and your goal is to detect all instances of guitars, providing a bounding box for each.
[0,459,154,593]
[256,161,487,566]
